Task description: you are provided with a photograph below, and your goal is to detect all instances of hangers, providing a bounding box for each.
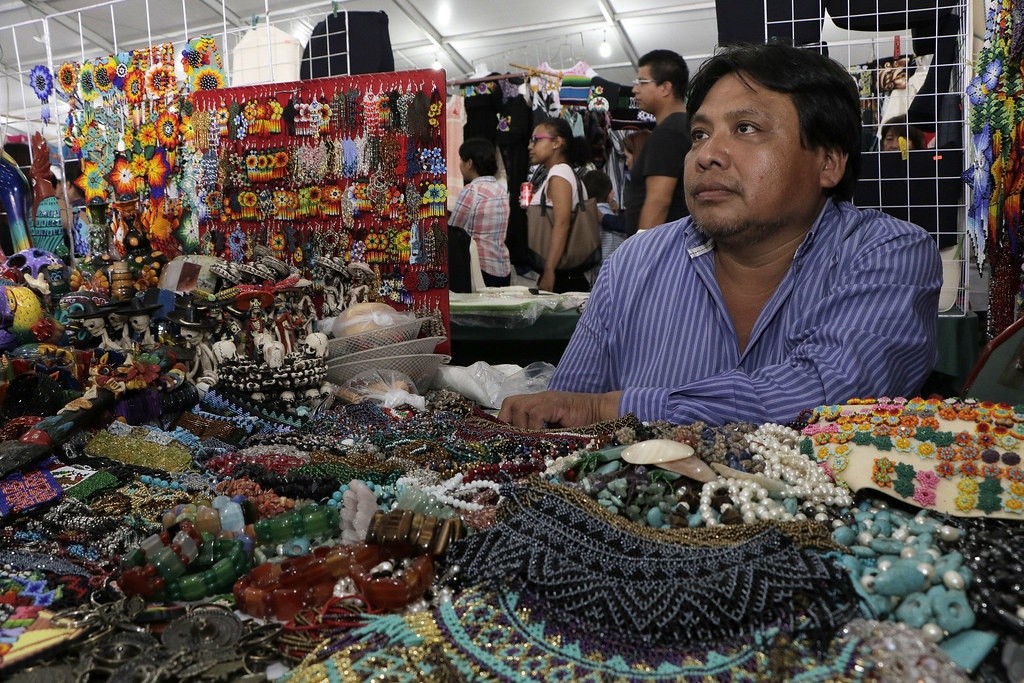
[468,29,608,87]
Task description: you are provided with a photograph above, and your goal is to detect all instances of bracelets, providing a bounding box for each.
[117,472,506,622]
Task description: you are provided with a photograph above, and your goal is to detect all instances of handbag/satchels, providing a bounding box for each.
[526,163,604,279]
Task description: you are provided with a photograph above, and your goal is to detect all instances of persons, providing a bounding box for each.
[447,48,692,294]
[851,91,963,249]
[0,142,91,250]
[497,44,942,432]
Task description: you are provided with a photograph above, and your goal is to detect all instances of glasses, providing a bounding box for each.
[632,76,657,87]
[529,134,560,142]
